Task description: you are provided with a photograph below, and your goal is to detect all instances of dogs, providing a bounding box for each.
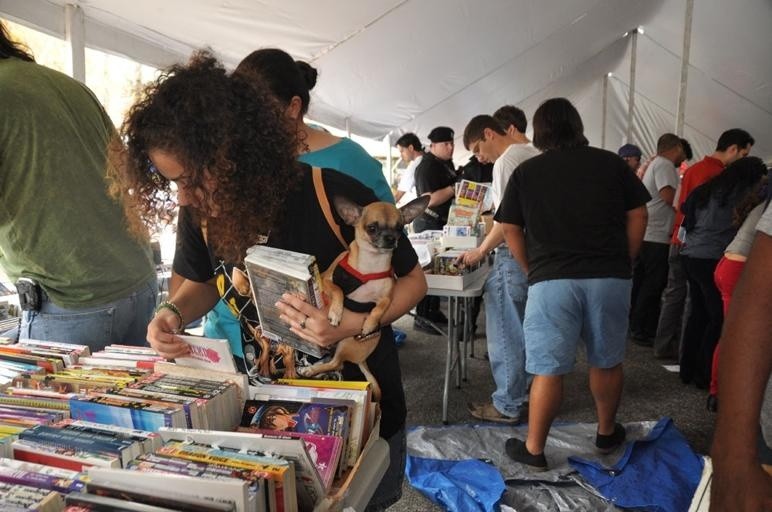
[296,193,430,403]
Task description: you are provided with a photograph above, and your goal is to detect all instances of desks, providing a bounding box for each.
[327,435,391,511]
[425,255,490,424]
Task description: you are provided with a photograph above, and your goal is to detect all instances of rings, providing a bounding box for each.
[299,316,310,329]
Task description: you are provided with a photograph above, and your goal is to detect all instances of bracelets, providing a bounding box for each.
[149,300,190,339]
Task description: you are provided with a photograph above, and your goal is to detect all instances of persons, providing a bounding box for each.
[168,44,399,379]
[392,98,772,512]
[0,20,158,345]
[99,40,429,512]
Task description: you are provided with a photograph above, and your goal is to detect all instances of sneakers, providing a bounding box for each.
[413,311,448,335]
[596,422,624,454]
[467,400,519,425]
[505,438,546,471]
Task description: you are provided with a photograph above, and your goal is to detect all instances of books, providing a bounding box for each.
[246,244,327,307]
[1,331,381,512]
[242,254,326,360]
